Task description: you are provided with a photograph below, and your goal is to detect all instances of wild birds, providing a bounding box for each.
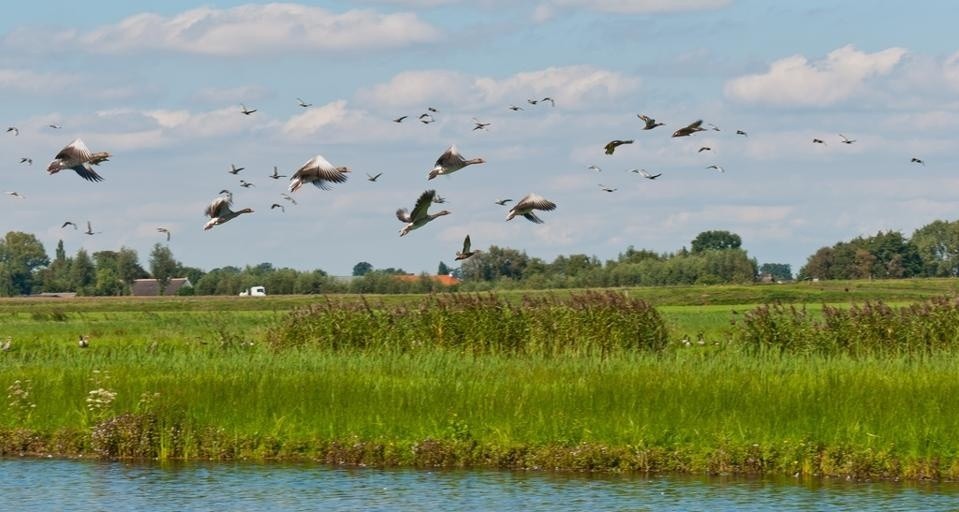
[78,335,89,348]
[418,107,437,125]
[679,329,734,347]
[395,190,452,238]
[0,336,12,353]
[427,144,487,180]
[587,113,748,191]
[366,171,383,183]
[239,102,257,116]
[496,198,513,207]
[392,115,408,124]
[271,203,285,211]
[280,192,297,204]
[526,97,554,106]
[203,198,254,233]
[218,189,235,202]
[288,154,353,193]
[269,165,287,180]
[812,133,855,144]
[238,178,257,190]
[452,234,480,261]
[297,98,313,107]
[227,163,244,175]
[6,123,113,236]
[507,104,525,112]
[911,157,924,166]
[470,116,490,133]
[506,193,556,225]
